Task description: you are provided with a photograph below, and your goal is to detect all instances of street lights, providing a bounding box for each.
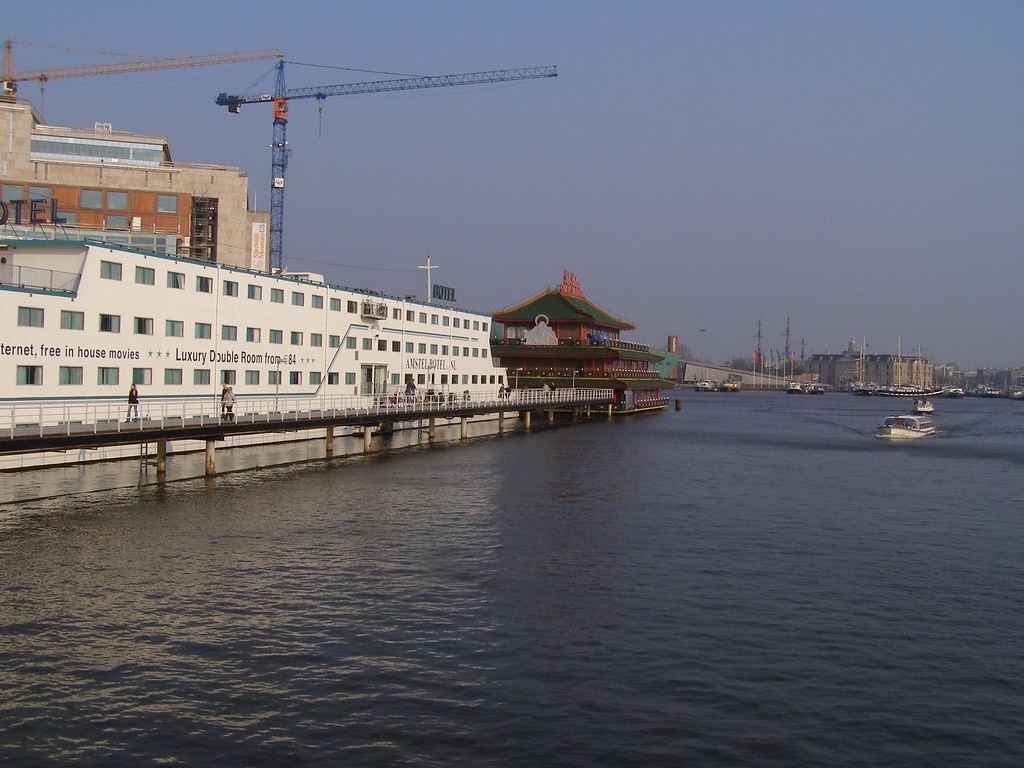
[699,329,707,379]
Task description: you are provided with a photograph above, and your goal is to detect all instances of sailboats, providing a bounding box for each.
[877,336,945,397]
[848,335,875,395]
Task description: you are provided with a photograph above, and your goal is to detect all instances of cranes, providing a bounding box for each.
[1,40,282,96]
[216,56,560,275]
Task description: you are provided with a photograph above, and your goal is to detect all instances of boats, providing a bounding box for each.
[808,385,825,395]
[877,415,936,438]
[786,383,803,392]
[940,388,964,398]
[717,380,741,392]
[965,385,1001,398]
[694,380,716,392]
[1009,390,1023,399]
[912,398,935,415]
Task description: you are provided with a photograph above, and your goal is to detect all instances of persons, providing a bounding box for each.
[220,383,235,421]
[543,383,549,399]
[499,384,506,402]
[505,385,511,402]
[125,383,139,423]
[405,379,416,395]
[551,382,556,399]
